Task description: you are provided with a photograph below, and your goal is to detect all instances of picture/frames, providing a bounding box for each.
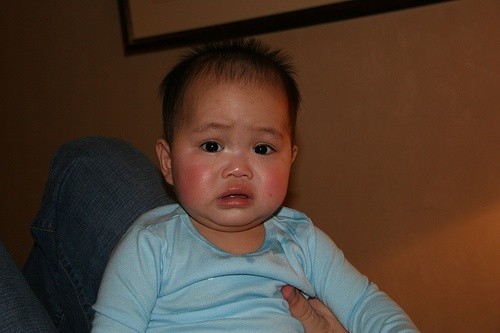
[119,0,449,54]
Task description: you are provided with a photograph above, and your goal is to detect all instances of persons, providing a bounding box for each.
[1,135,350,332]
[88,31,424,333]
